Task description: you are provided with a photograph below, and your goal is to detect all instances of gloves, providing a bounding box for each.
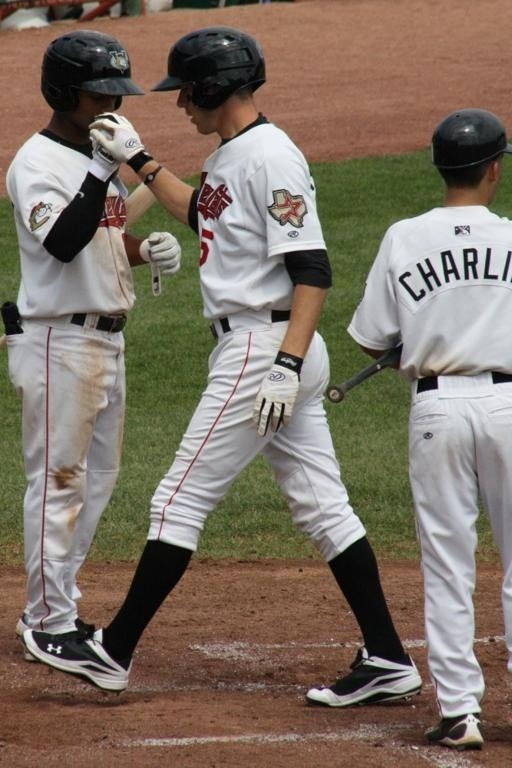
[252,350,304,438]
[88,111,145,200]
[139,231,182,296]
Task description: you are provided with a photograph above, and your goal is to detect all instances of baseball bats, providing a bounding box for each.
[326,341,404,404]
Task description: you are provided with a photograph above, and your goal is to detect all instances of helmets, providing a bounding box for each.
[150,27,267,110]
[431,108,512,168]
[41,30,145,113]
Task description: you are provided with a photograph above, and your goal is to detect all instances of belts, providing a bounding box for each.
[208,309,294,339]
[70,312,128,334]
[416,371,512,395]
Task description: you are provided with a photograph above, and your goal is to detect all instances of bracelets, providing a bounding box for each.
[141,165,164,185]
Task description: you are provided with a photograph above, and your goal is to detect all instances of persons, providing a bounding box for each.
[344,109,512,751]
[20,29,423,709]
[0,31,182,663]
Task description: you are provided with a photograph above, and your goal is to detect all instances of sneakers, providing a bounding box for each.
[307,649,422,706]
[424,713,485,750]
[15,613,133,690]
